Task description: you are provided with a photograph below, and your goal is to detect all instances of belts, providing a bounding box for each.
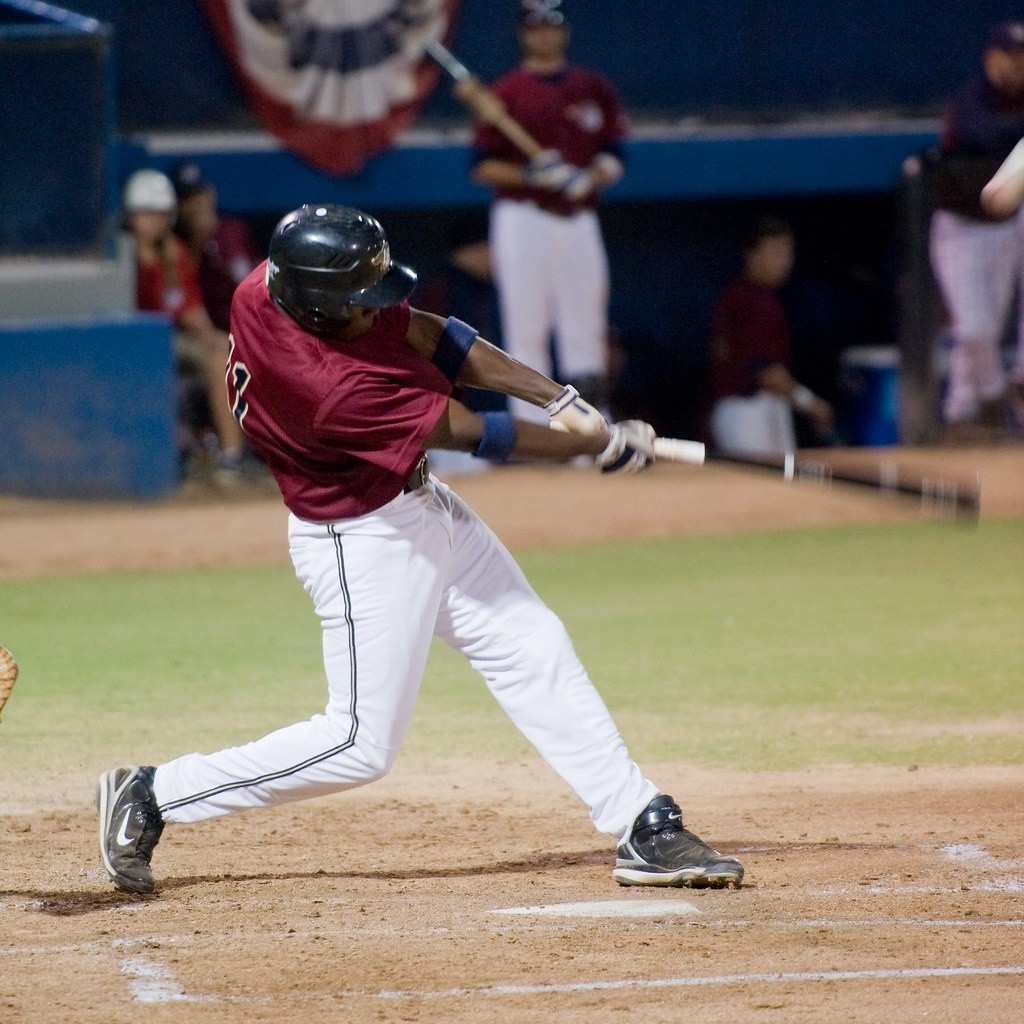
[400,456,432,495]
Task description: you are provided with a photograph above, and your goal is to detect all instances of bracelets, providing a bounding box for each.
[439,311,518,459]
[795,387,812,409]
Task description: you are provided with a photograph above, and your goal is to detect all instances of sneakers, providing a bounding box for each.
[94,765,167,895]
[614,795,746,890]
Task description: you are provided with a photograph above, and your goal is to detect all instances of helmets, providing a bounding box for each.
[264,202,420,338]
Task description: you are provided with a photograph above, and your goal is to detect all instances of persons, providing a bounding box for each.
[467,2,640,467]
[930,24,1024,436]
[97,204,744,895]
[707,223,854,456]
[124,158,259,474]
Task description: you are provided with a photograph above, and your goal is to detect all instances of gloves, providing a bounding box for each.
[593,426,655,478]
[523,147,593,203]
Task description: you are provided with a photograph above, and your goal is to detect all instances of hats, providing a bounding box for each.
[988,21,1024,45]
[123,173,174,214]
[511,0,576,27]
[169,162,205,198]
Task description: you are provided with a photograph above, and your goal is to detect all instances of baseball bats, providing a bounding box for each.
[647,432,990,537]
